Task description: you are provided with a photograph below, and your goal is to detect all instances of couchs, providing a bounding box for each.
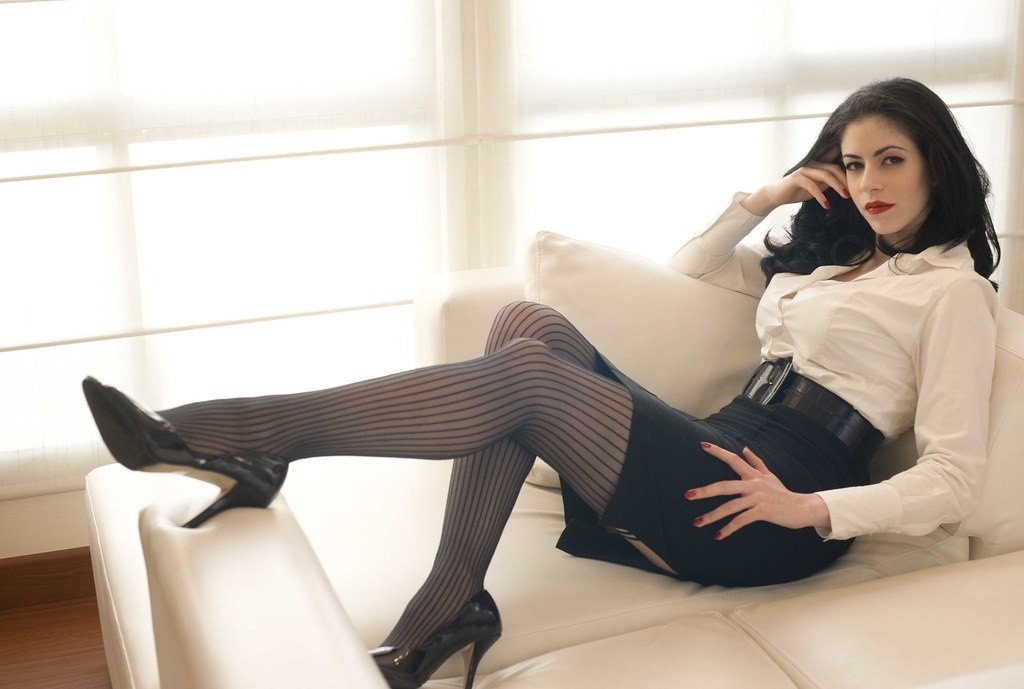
[83,268,1024,689]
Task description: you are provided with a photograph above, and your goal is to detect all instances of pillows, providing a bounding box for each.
[520,225,974,560]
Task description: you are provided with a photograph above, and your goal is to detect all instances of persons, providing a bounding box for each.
[80,75,1002,689]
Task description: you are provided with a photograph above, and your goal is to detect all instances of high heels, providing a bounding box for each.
[82,376,288,527]
[372,591,501,689]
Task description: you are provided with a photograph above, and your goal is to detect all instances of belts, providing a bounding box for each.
[742,360,884,459]
